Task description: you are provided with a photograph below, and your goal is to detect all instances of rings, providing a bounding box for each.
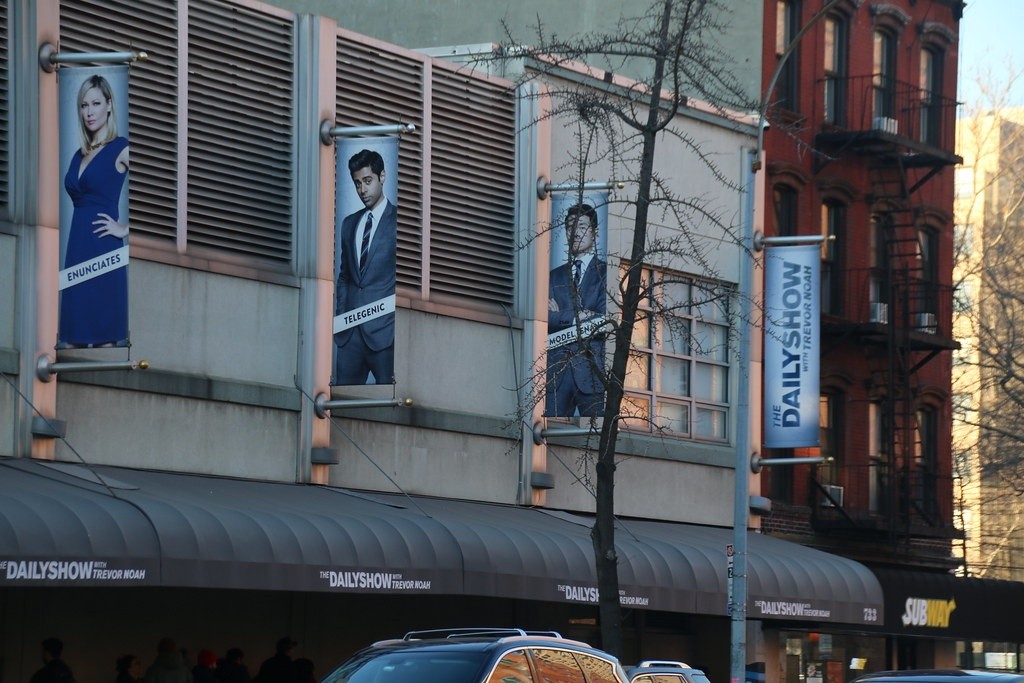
[104,226,107,230]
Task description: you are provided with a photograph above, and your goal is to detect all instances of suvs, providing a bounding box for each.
[319,627,630,683]
[620,660,711,683]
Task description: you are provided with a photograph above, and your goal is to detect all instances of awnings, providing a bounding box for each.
[0,457,885,628]
[761,564,1023,643]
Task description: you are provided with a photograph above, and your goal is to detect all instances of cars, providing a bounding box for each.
[847,669,1023,683]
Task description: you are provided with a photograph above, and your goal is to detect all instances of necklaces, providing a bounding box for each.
[87,141,101,151]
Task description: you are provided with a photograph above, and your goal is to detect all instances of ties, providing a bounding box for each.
[359,213,373,275]
[573,259,583,293]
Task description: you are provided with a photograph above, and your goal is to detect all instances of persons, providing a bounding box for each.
[57,74,129,349]
[542,203,609,417]
[334,148,399,386]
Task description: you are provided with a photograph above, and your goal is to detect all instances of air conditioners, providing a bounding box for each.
[820,483,845,507]
[871,116,898,136]
[914,312,937,333]
[868,302,888,324]
